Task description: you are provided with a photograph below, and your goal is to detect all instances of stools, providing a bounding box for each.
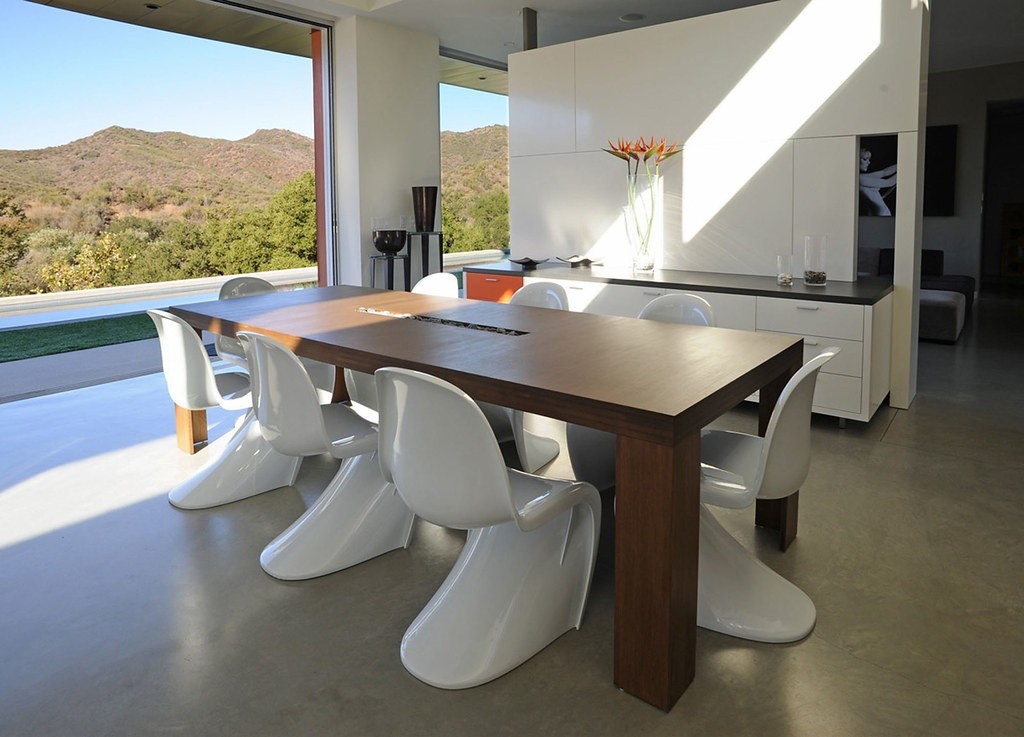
[924,275,975,321]
[919,289,966,346]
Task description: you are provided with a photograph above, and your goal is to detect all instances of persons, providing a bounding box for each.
[858,149,897,216]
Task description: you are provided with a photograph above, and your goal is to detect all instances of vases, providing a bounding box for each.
[803,236,828,286]
[372,230,407,256]
[412,186,438,232]
[633,252,656,275]
[776,256,794,286]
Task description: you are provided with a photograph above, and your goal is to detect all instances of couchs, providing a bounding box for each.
[857,247,944,289]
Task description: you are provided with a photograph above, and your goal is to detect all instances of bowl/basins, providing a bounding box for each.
[373,230,407,256]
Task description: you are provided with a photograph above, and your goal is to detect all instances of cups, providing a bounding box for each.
[412,187,437,232]
[806,235,825,287]
[776,255,793,286]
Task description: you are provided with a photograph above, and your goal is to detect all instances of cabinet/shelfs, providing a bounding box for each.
[756,291,894,422]
[523,276,756,331]
[467,272,523,304]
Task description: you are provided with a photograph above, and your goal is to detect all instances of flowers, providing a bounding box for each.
[601,135,685,252]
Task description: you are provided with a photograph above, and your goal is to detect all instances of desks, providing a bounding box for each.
[169,284,804,715]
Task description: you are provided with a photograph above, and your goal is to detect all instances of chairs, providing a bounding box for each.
[344,272,459,412]
[236,331,417,581]
[373,367,600,692]
[614,347,843,644]
[146,309,304,510]
[566,294,716,492]
[475,279,570,473]
[215,276,277,372]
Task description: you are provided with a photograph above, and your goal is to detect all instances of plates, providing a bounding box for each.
[556,255,594,267]
[509,257,550,271]
